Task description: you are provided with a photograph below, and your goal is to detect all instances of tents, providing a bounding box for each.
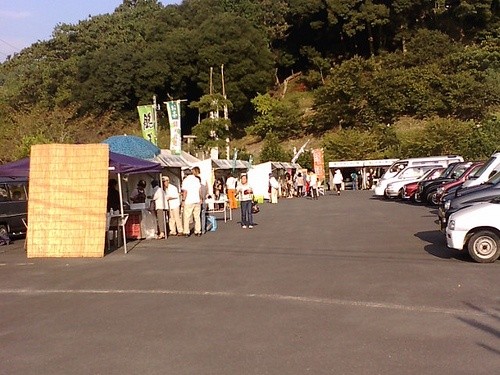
[115,149,211,242]
[0,145,164,254]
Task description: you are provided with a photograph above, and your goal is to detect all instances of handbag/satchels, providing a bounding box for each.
[251,201,260,214]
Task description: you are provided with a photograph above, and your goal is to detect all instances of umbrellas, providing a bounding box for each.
[99,132,160,159]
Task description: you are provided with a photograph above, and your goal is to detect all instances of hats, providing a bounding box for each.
[162,176,169,181]
[137,179,147,188]
[335,169,341,174]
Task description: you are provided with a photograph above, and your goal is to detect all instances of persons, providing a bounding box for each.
[108,167,209,238]
[233,174,256,229]
[333,169,344,196]
[226,172,238,210]
[205,194,215,215]
[12,190,22,200]
[349,166,374,192]
[270,170,320,204]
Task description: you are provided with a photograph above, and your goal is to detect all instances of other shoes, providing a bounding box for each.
[248,225,253,229]
[242,225,247,229]
[159,232,202,239]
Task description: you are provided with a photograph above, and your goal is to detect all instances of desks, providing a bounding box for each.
[205,200,233,222]
[106,214,128,253]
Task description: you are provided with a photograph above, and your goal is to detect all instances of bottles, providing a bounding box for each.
[110,208,113,216]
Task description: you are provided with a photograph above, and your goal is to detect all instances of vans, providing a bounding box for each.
[372,151,500,263]
[0,178,29,246]
